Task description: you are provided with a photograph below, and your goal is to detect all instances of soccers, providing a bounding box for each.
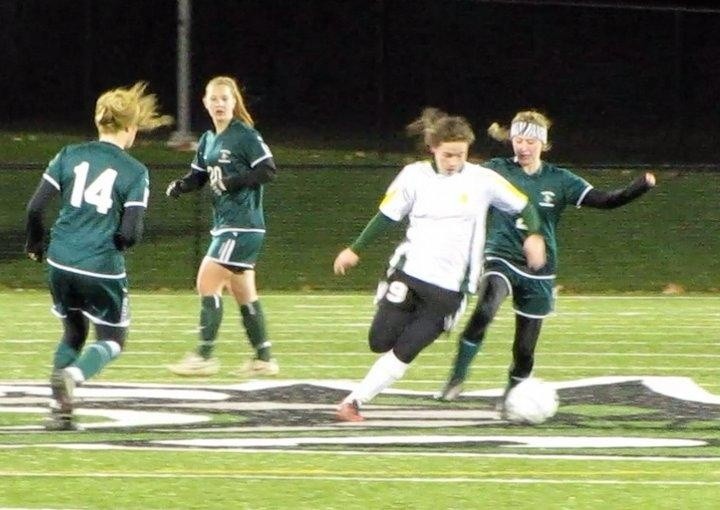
[506,377,559,425]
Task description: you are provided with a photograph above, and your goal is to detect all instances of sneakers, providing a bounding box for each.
[231,355,280,377]
[43,371,85,434]
[331,396,366,424]
[433,383,464,402]
[167,353,218,376]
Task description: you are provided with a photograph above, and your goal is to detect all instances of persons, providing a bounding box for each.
[437,109,660,406]
[163,75,283,378]
[332,107,548,423]
[22,76,176,429]
